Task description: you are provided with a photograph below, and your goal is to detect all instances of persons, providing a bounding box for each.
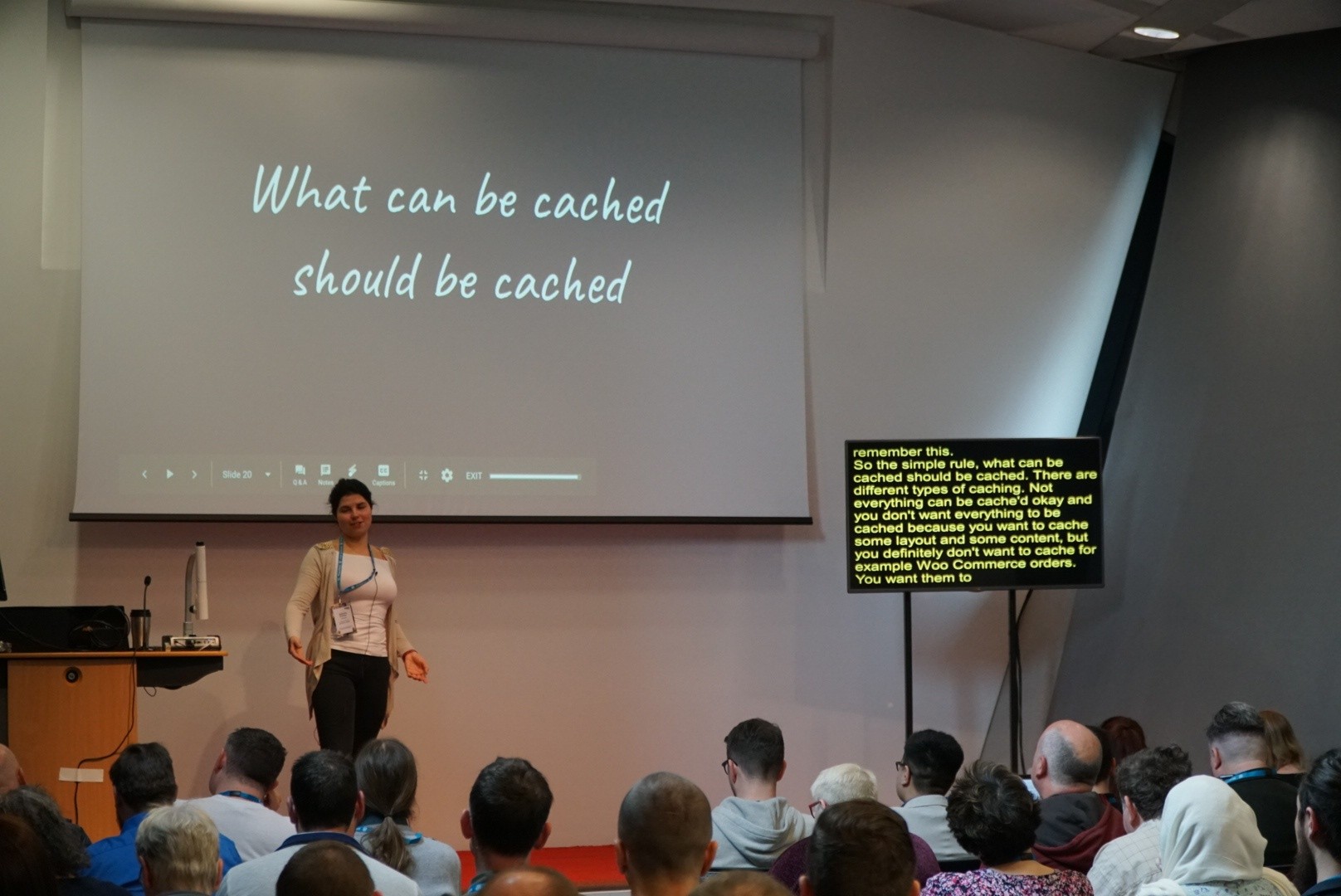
[921,759,1093,896]
[1086,744,1193,896]
[801,796,921,896]
[772,764,940,896]
[284,479,428,760]
[174,727,297,862]
[461,757,584,896]
[214,750,426,896]
[0,745,93,847]
[617,771,718,896]
[135,806,223,895]
[275,839,384,896]
[1024,719,1126,879]
[351,739,461,896]
[1204,702,1299,874]
[79,741,242,896]
[1085,710,1341,896]
[0,787,132,896]
[690,717,816,896]
[888,730,983,862]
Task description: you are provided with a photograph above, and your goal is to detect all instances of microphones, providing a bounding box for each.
[138,576,151,651]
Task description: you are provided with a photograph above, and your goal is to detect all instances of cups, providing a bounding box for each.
[131,609,151,651]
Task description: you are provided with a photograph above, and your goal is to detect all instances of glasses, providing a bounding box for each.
[896,762,912,775]
[722,758,737,775]
[809,802,820,819]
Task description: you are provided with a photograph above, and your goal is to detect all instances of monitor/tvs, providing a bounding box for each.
[843,437,1106,594]
